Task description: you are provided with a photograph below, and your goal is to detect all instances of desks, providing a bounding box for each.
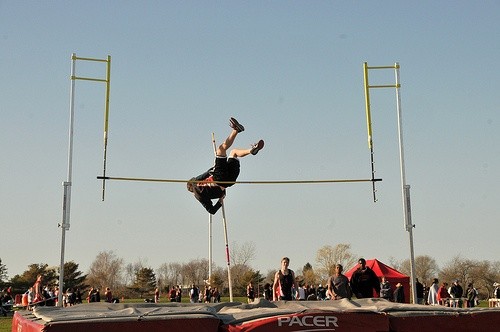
[489,299,500,307]
[439,298,468,308]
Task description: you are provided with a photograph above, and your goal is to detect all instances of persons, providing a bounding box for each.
[466,283,479,308]
[349,258,381,299]
[189,284,221,303]
[0,285,118,307]
[32,274,46,309]
[327,264,350,300]
[427,278,440,305]
[264,280,328,301]
[273,257,300,301]
[393,283,405,303]
[247,281,254,303]
[381,280,393,302]
[169,285,182,302]
[494,285,500,307]
[436,282,452,305]
[448,280,463,308]
[186,117,264,214]
[155,288,159,304]
[415,278,423,304]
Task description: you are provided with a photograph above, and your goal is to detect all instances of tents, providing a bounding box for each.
[343,259,412,301]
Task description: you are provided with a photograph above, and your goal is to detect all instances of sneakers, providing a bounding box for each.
[250,140,264,155]
[229,117,244,132]
